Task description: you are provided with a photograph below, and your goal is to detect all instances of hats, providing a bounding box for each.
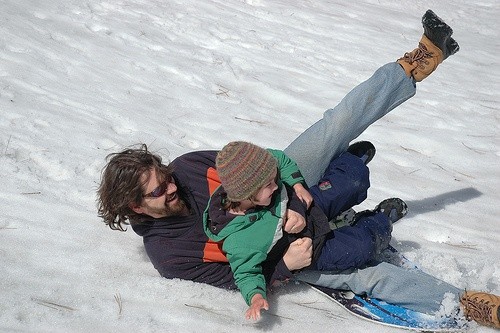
[215,141,278,202]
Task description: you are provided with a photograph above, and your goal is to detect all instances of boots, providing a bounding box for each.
[396,10,459,82]
[458,288,500,329]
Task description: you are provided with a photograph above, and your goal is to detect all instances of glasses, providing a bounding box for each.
[143,173,172,197]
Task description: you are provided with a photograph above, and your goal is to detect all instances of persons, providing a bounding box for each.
[203,141,408,322]
[97,9,500,330]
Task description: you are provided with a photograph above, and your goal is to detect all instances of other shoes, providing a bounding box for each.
[347,141,376,164]
[373,197,408,224]
